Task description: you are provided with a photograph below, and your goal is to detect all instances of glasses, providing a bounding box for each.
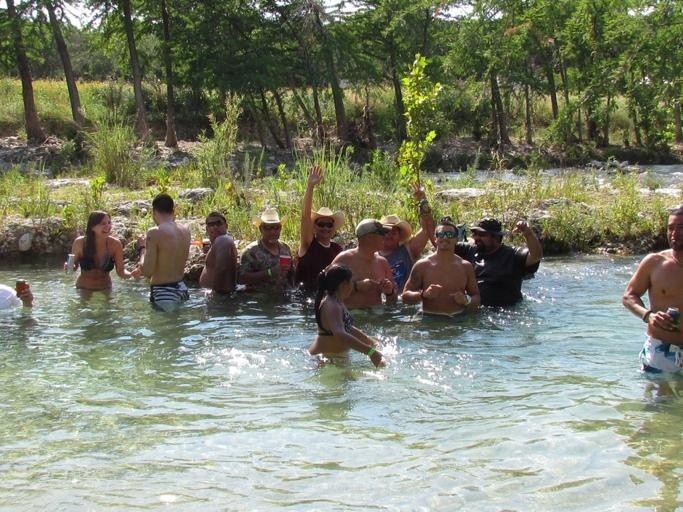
[263,225,281,230]
[207,221,225,227]
[435,231,457,239]
[315,222,334,228]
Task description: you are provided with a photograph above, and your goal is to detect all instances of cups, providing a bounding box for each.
[279,255,291,273]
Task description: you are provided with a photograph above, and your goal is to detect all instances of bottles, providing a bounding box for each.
[202,239,211,256]
[66,253,75,275]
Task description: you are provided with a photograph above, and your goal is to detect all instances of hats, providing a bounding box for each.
[470,218,502,234]
[311,207,346,233]
[380,214,412,242]
[355,219,383,239]
[252,208,288,232]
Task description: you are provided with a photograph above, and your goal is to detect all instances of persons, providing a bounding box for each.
[237,208,296,291]
[333,218,397,308]
[402,222,481,317]
[135,192,193,303]
[63,210,143,295]
[309,265,383,367]
[0,285,35,309]
[411,181,544,307]
[198,211,238,294]
[620,204,683,376]
[293,165,347,286]
[376,214,429,292]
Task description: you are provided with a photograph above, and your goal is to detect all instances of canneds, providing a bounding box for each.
[67,254,75,276]
[666,307,681,332]
[16,279,25,297]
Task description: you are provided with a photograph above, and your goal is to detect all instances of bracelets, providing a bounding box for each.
[419,290,424,299]
[384,289,394,296]
[642,310,653,323]
[367,348,375,357]
[353,281,359,292]
[267,268,272,276]
[138,245,145,251]
[416,199,431,215]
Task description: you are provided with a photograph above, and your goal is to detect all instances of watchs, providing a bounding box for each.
[465,295,471,306]
